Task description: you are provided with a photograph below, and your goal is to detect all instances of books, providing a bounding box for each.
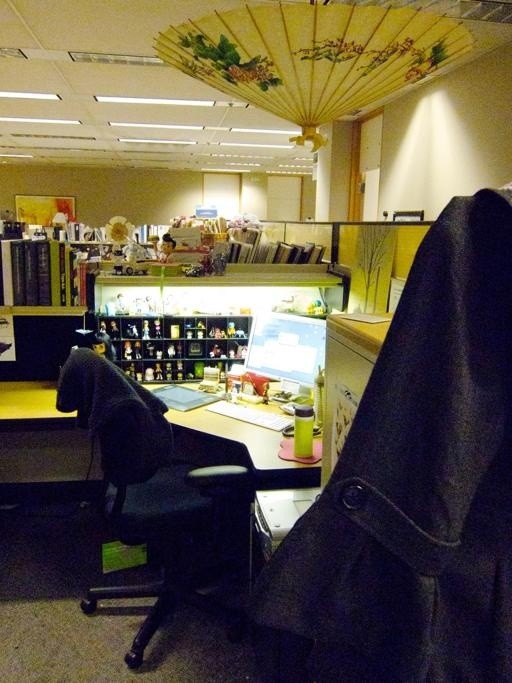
[131,224,170,244]
[170,217,325,264]
[150,384,223,412]
[1,222,108,307]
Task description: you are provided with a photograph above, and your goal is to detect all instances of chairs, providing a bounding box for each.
[58,348,250,667]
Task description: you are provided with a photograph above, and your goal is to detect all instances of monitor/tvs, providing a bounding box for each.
[244,312,326,403]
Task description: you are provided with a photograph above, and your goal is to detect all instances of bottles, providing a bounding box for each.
[294,407,313,457]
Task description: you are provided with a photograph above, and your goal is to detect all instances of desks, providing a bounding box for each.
[0,370,322,592]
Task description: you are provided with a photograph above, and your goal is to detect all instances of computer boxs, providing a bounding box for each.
[75,481,195,576]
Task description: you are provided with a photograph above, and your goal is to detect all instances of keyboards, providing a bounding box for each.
[205,400,294,433]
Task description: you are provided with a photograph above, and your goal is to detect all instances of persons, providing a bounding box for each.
[160,234,176,263]
[99,319,248,381]
[112,250,125,275]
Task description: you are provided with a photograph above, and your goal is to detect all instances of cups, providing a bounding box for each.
[60,230,65,240]
[204,368,219,383]
[313,373,324,425]
